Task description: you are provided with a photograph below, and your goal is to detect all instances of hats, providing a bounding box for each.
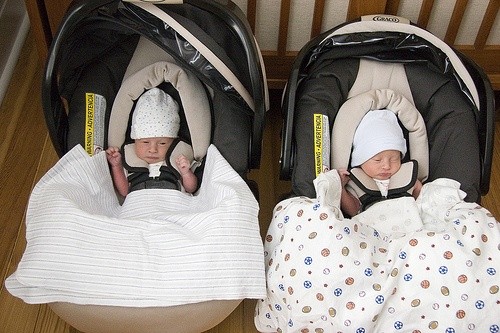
[351,110,407,167]
[130,87,181,139]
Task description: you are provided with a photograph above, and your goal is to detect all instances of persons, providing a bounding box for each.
[337,110,425,240]
[103,88,198,222]
